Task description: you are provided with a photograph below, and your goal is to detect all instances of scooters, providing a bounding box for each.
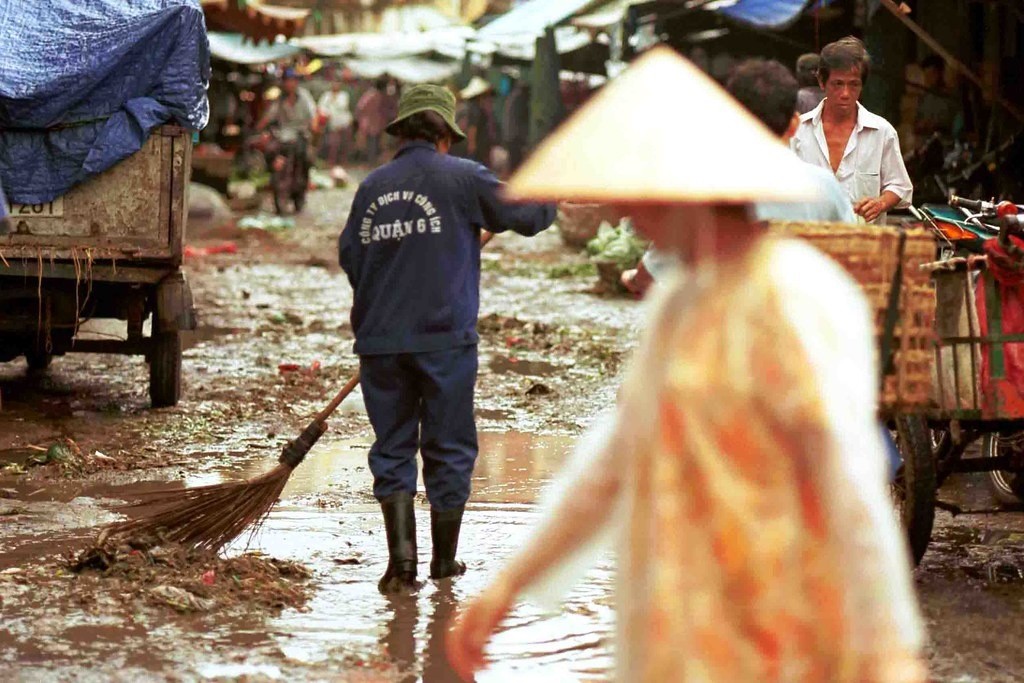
[262,127,312,216]
[876,195,1024,573]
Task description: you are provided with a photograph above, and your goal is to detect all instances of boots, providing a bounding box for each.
[381,492,418,583]
[431,507,466,578]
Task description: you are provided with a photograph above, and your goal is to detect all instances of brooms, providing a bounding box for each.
[115,366,362,553]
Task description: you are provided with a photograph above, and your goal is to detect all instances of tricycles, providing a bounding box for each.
[0,124,196,407]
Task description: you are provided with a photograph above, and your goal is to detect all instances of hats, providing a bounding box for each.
[282,68,304,77]
[504,43,824,202]
[383,84,467,144]
[795,54,822,76]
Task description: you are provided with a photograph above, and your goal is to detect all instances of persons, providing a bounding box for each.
[338,84,555,592]
[442,37,929,683]
[252,67,401,184]
